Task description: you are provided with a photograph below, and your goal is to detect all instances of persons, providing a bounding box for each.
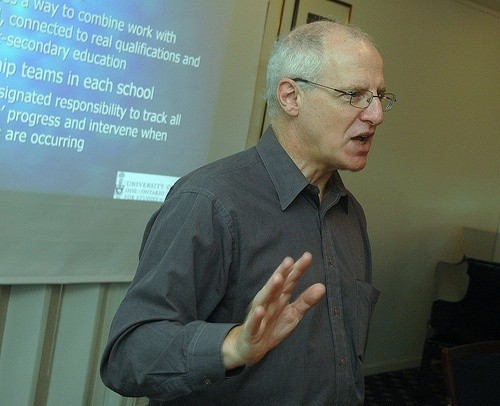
[99,20,389,406]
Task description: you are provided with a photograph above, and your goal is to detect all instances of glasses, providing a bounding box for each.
[292,77,398,112]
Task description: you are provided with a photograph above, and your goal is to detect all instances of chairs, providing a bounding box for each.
[441,340,500,406]
